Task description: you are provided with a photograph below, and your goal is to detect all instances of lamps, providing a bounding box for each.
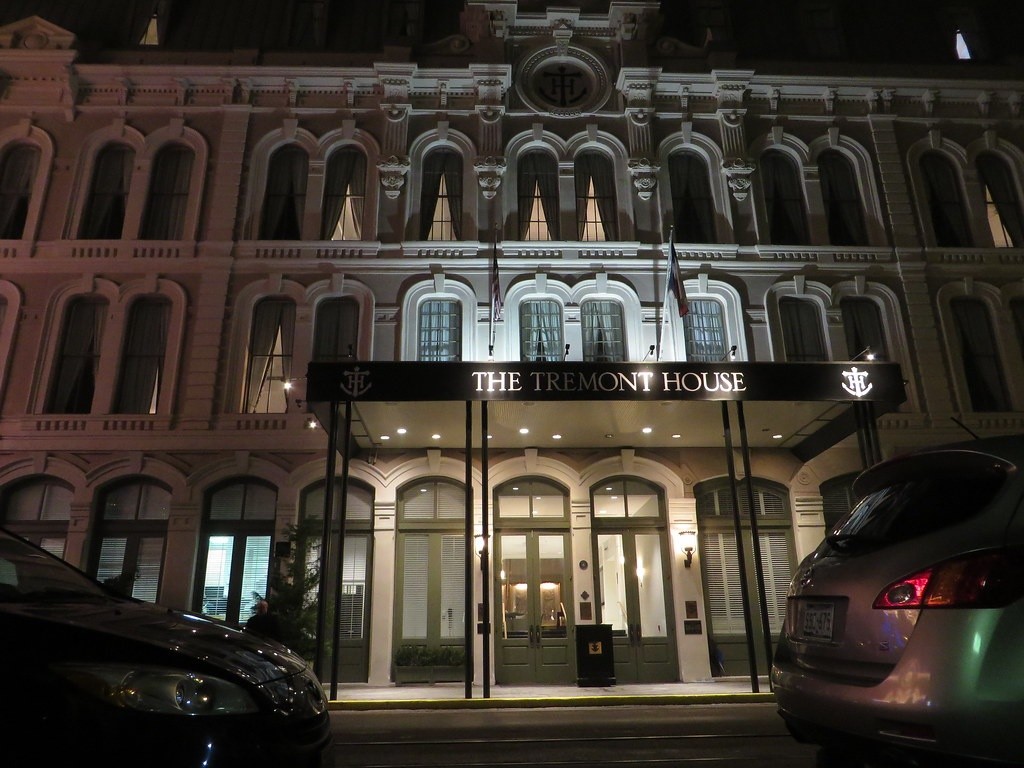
[347,344,358,361]
[474,534,491,571]
[643,345,655,361]
[721,346,737,361]
[489,345,493,356]
[678,531,696,568]
[564,344,570,361]
[295,399,306,408]
[850,345,873,361]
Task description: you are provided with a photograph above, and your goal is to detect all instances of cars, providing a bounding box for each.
[0,526,334,767]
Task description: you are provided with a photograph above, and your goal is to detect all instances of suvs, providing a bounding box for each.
[767,431,1024,767]
